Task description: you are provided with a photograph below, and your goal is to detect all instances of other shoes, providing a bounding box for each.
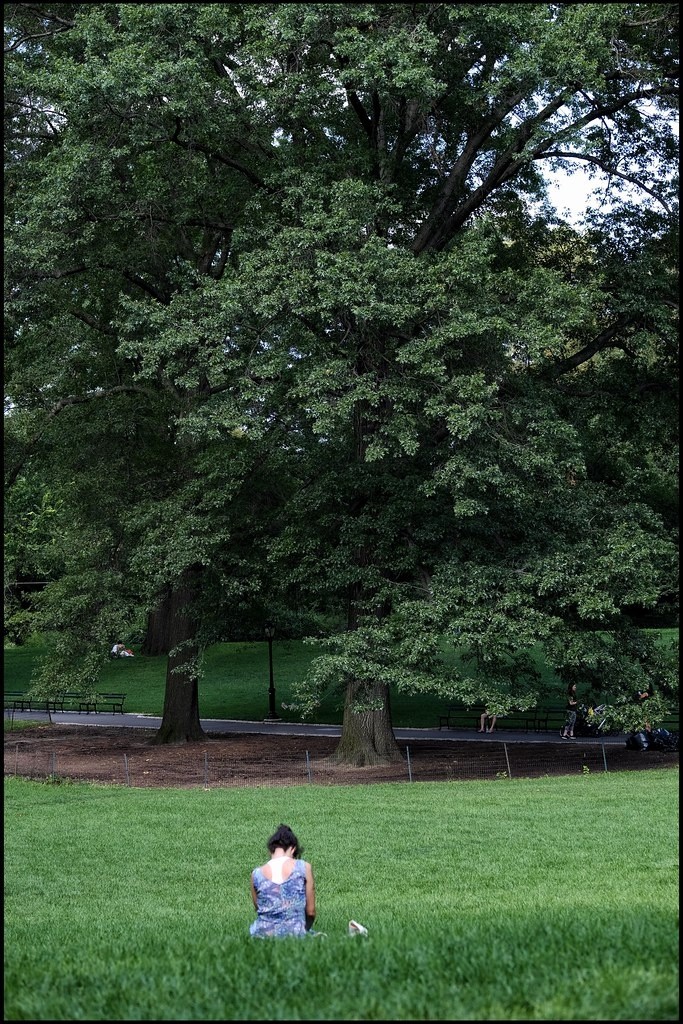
[561,735,576,740]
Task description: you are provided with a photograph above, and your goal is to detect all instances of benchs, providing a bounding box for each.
[437,704,568,735]
[3,691,127,716]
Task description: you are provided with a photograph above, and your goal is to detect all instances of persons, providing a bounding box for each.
[478,709,503,733]
[561,682,580,739]
[640,682,654,732]
[250,823,327,940]
[110,640,125,659]
